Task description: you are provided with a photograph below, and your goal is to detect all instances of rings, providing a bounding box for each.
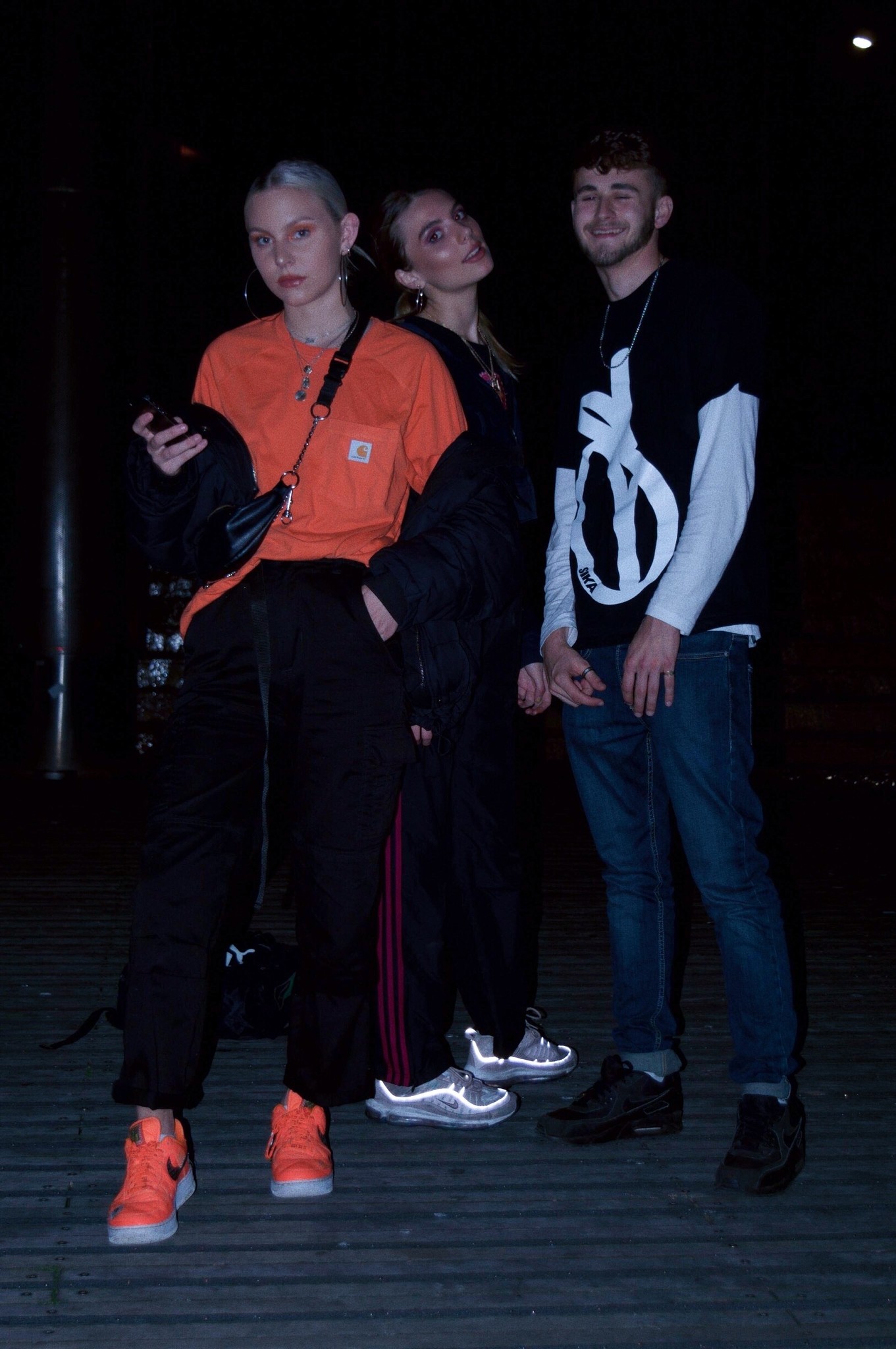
[664,670,674,676]
[582,667,594,677]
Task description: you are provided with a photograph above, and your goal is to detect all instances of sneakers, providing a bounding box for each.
[363,1065,519,1129]
[462,1008,578,1088]
[265,1087,335,1197]
[108,1116,195,1245]
[717,1091,808,1195]
[538,1056,685,1140]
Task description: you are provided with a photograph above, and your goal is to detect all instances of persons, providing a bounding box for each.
[539,128,806,1201]
[107,161,492,1245]
[363,184,578,1127]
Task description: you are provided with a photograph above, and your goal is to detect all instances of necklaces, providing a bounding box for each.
[599,254,665,369]
[422,308,502,391]
[288,309,360,400]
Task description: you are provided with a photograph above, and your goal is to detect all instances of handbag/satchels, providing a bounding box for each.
[192,481,291,584]
[115,924,297,1042]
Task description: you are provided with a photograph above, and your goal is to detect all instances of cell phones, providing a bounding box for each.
[129,395,190,442]
